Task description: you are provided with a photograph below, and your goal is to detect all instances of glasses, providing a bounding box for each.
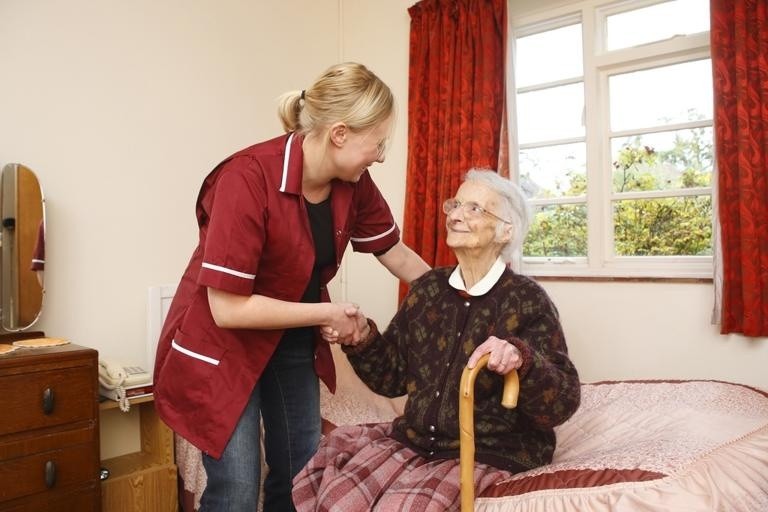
[442,197,511,226]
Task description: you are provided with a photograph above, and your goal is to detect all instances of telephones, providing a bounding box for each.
[98,362,152,387]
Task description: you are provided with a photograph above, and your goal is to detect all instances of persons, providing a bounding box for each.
[151,61,434,512]
[314,168,581,511]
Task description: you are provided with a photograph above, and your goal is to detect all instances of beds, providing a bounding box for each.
[145,283,767,512]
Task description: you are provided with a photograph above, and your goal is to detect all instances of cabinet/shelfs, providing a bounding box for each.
[1,340,102,512]
[101,394,179,512]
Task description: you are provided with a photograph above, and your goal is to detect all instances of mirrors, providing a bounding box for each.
[0,162,46,333]
